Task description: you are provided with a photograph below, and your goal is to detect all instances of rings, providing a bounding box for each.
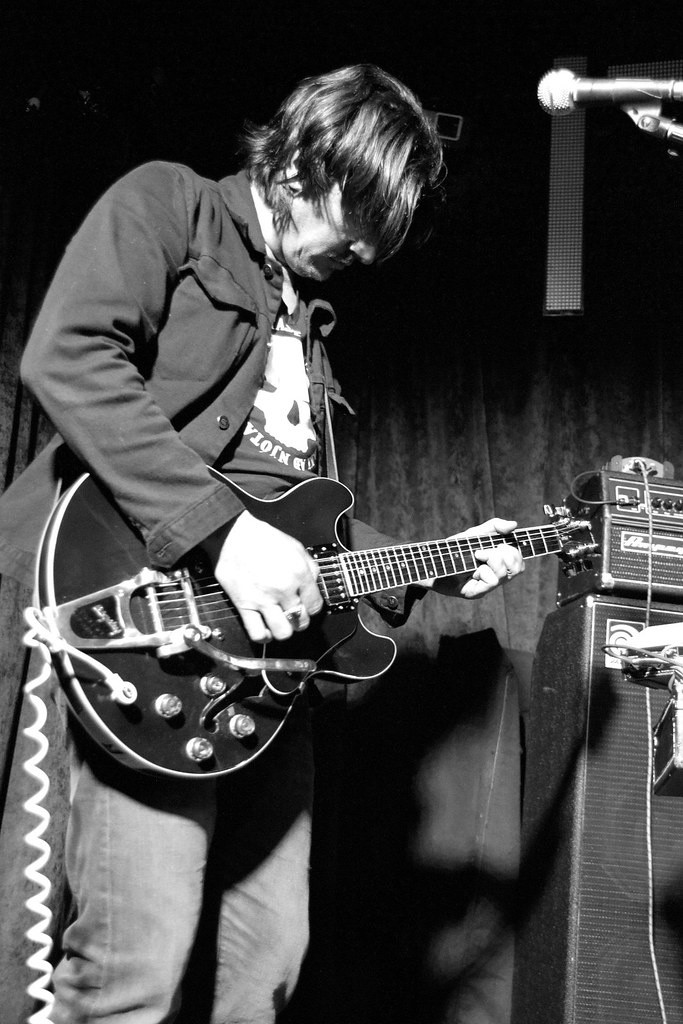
[282,604,304,619]
[507,562,522,576]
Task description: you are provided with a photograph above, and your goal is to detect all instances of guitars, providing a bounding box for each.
[34,461,603,785]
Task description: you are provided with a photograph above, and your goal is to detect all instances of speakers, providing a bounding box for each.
[510,593,683,1024]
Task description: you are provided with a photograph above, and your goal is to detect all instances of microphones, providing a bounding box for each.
[538,68,683,116]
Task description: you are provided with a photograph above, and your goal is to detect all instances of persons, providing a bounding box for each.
[0,64,527,1024]
